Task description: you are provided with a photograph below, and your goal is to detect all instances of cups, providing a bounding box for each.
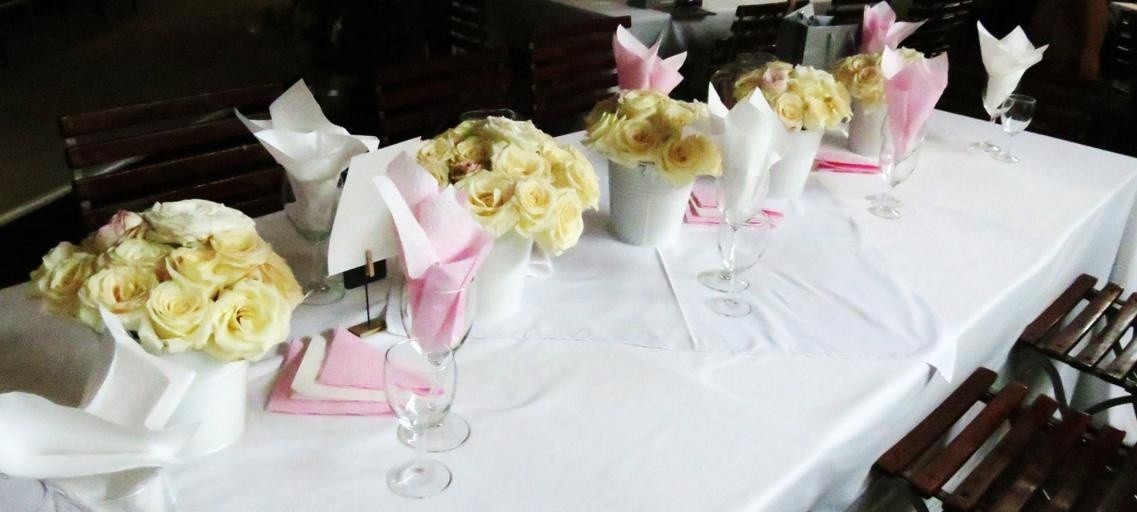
[969,83,1019,155]
[993,93,1036,165]
[708,206,772,317]
[868,138,919,218]
[397,278,476,453]
[382,337,457,501]
[282,179,345,308]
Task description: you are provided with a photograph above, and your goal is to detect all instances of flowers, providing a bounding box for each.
[830,47,926,114]
[581,87,723,184]
[735,62,854,133]
[31,199,302,361]
[419,116,600,257]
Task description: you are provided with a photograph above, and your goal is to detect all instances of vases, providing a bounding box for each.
[768,129,822,194]
[173,361,252,452]
[604,159,694,244]
[466,237,532,318]
[847,99,890,156]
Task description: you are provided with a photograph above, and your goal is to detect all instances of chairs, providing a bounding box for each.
[873,370,1135,511]
[829,0,890,45]
[713,2,809,63]
[901,0,970,57]
[528,16,631,129]
[1019,276,1136,422]
[1019,10,1135,118]
[55,83,297,232]
[375,42,514,148]
